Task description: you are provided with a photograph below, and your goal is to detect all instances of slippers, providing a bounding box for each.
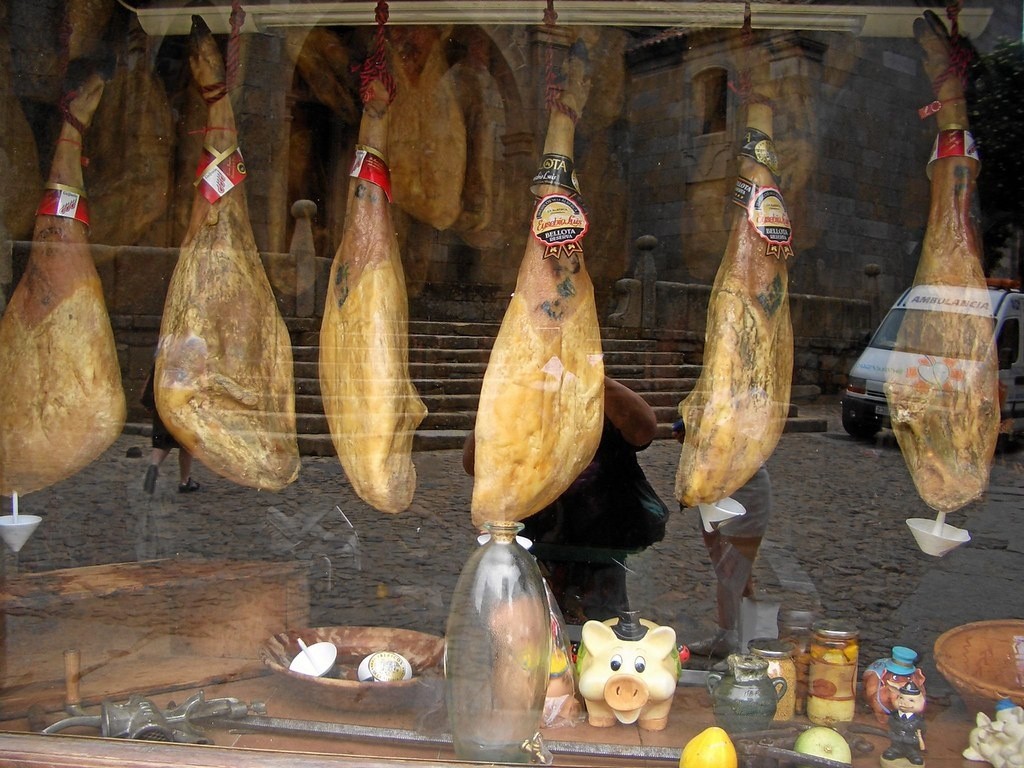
[179,478,200,492]
[144,463,158,495]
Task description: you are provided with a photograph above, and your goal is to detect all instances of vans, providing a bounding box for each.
[841,277,1024,448]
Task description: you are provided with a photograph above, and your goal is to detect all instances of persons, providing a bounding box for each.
[462,369,668,627]
[145,306,208,493]
[679,396,771,656]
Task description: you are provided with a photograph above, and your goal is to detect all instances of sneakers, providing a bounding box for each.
[688,628,739,660]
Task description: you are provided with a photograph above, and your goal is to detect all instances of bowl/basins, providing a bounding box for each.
[933,619,1024,723]
[258,626,442,711]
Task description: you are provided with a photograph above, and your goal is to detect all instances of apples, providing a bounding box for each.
[795,727,851,768]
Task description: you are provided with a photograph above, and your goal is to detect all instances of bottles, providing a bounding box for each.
[776,607,819,714]
[708,652,788,732]
[748,637,797,723]
[807,619,860,725]
[443,525,553,764]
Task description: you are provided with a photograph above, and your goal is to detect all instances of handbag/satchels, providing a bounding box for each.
[141,372,155,412]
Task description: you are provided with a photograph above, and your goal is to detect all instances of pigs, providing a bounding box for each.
[575,615,681,732]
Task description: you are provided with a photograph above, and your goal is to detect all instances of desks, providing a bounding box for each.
[1,655,995,767]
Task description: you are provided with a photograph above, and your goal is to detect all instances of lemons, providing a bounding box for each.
[679,727,738,768]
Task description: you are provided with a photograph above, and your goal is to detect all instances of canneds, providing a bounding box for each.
[807,623,859,726]
